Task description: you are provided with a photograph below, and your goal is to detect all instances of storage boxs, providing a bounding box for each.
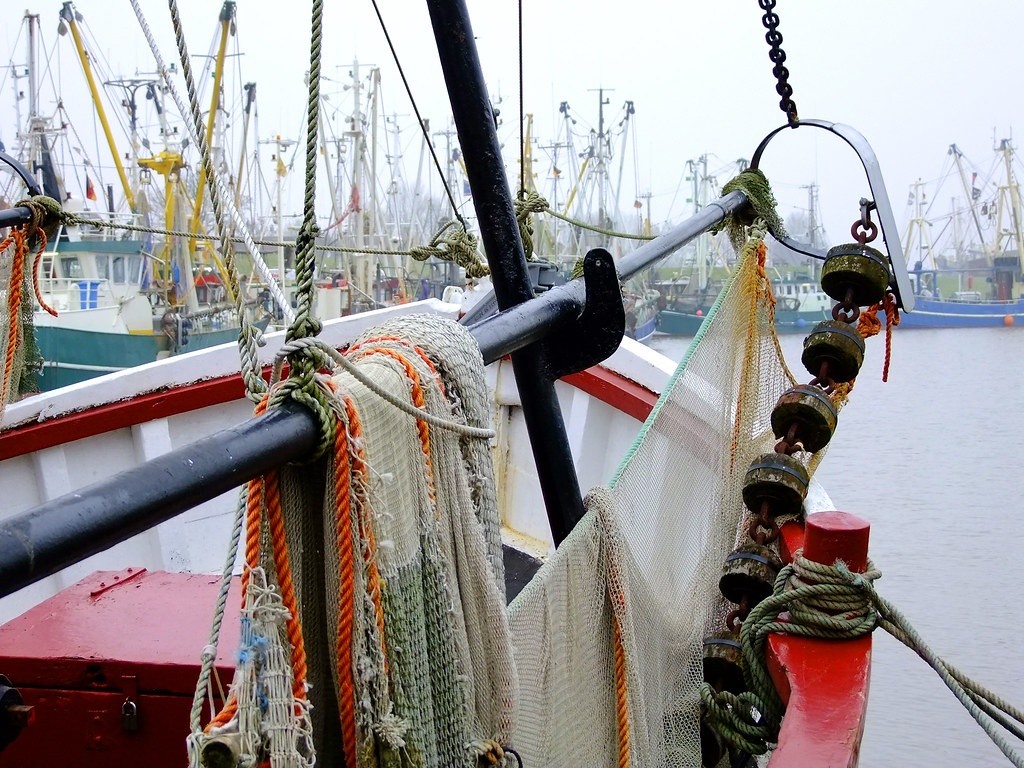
[0,570,243,768]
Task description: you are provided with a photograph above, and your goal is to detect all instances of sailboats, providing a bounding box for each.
[0,0,661,403]
[658,154,840,336]
[877,125,1024,330]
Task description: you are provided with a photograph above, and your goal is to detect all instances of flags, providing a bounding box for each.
[86,175,97,200]
